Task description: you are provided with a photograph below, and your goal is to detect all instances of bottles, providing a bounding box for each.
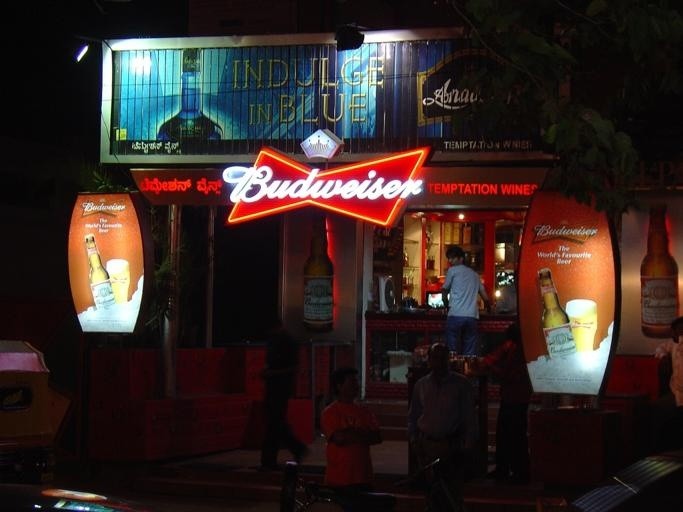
[302,218,334,332]
[83,233,117,308]
[537,270,575,360]
[159,50,223,140]
[641,208,679,338]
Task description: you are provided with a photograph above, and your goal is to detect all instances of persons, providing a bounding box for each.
[407,341,476,512]
[441,245,491,356]
[322,367,384,510]
[648,316,683,450]
[469,322,530,484]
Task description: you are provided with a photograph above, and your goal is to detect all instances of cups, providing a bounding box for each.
[565,298,597,355]
[105,259,129,303]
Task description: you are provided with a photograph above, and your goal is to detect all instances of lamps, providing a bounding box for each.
[64,34,91,63]
[333,17,364,51]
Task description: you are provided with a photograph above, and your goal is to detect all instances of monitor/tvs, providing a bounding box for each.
[427,290,450,308]
